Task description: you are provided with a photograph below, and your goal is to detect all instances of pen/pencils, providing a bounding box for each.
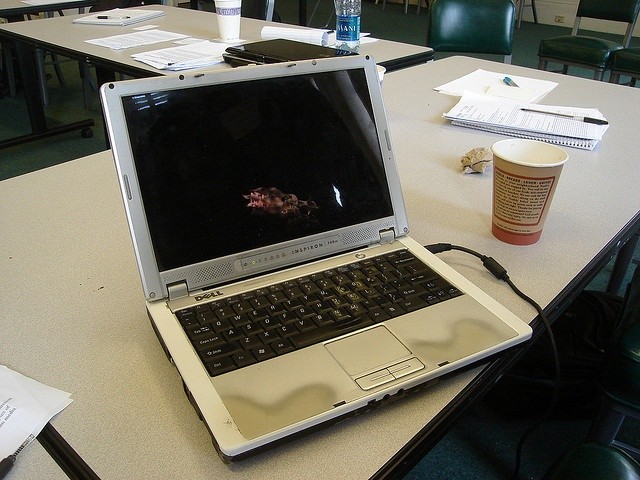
[503,74,520,90]
[92,14,133,20]
[519,105,608,130]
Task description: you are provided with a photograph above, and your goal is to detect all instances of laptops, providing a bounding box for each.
[98,55,535,464]
[222,38,359,68]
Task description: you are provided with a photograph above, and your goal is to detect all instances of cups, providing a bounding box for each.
[489,137,571,246]
[213,1,243,41]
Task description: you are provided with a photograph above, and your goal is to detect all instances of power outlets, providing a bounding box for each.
[554,16,565,23]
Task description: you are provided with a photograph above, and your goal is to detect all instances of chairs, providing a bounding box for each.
[537,0,640,81]
[607,47,640,84]
[588,314,640,450]
[428,1,515,65]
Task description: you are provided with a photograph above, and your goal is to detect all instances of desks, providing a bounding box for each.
[1,55,640,478]
[0,3,435,152]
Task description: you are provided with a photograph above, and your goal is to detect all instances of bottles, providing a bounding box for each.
[333,0,362,53]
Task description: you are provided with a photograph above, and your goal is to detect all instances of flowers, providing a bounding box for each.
[240,185,319,231]
